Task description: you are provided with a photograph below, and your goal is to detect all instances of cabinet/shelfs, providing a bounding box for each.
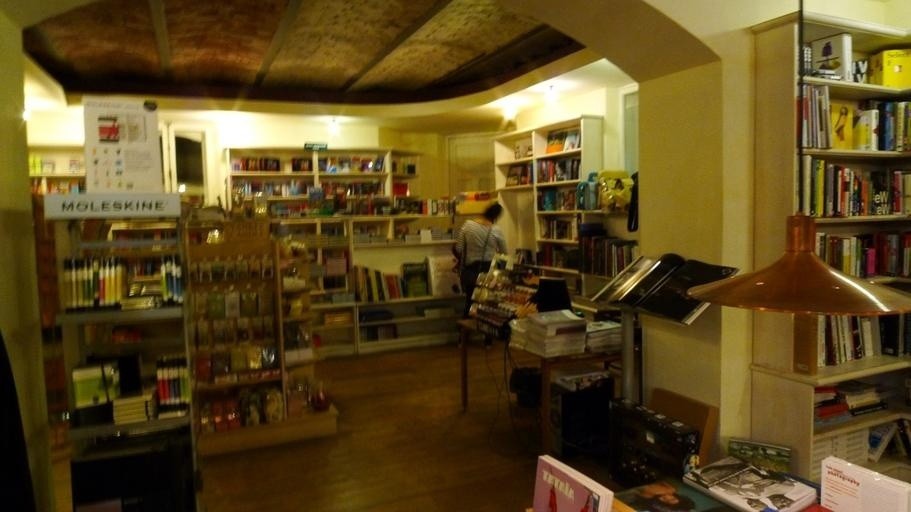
[745,10,910,487]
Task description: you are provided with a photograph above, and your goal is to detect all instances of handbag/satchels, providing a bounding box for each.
[576,173,599,210]
[459,261,482,283]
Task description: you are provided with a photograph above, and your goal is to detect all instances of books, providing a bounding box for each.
[802,85,911,152]
[803,155,911,217]
[867,419,911,463]
[60,215,189,429]
[620,446,906,511]
[530,453,612,512]
[818,313,911,363]
[355,255,464,302]
[801,34,855,82]
[814,379,894,423]
[536,170,638,279]
[500,126,584,183]
[814,232,911,278]
[338,198,459,216]
[228,152,417,196]
[505,301,630,357]
[29,155,81,192]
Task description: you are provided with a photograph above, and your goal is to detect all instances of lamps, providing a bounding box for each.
[687,1,910,319]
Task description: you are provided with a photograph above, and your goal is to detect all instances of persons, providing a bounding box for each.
[452,201,508,352]
